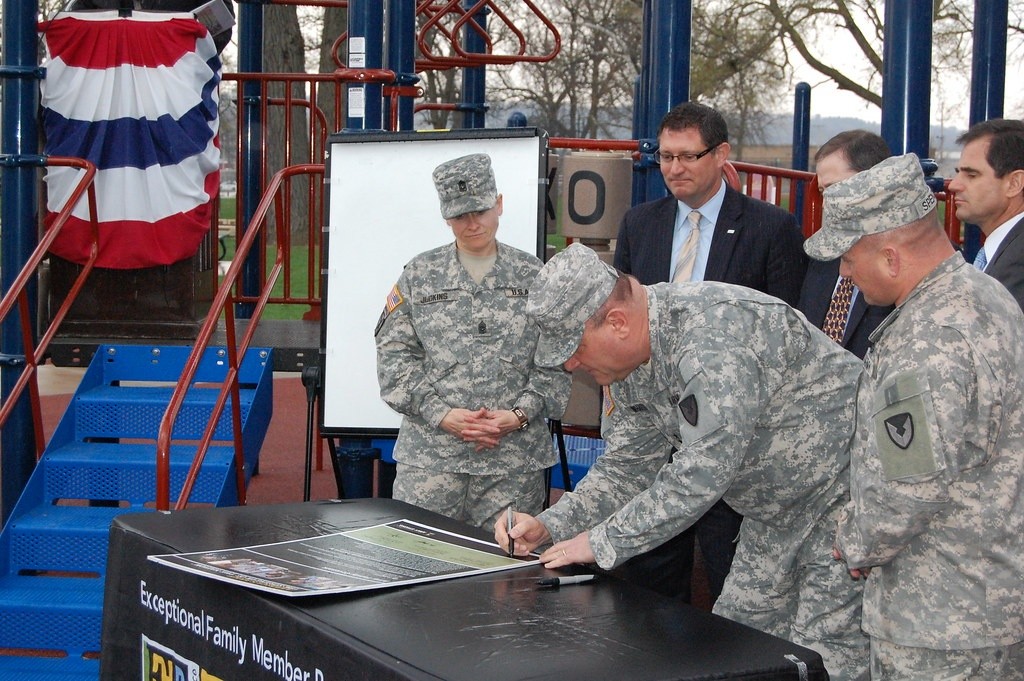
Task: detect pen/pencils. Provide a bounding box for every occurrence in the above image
[507,507,514,558]
[534,574,598,586]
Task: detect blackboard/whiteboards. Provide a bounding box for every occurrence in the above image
[321,127,549,439]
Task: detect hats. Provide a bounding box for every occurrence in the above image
[525,242,619,368]
[432,153,497,219]
[803,153,937,261]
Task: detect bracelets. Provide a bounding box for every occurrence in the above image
[509,406,530,432]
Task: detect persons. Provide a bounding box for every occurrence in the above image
[373,151,571,549]
[494,100,1024,681]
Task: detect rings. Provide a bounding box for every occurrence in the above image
[563,549,567,556]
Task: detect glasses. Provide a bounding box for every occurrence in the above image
[653,143,721,163]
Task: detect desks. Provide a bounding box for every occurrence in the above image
[96,497,829,681]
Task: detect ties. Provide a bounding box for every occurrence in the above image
[671,210,702,282]
[822,277,854,346]
[973,246,988,271]
